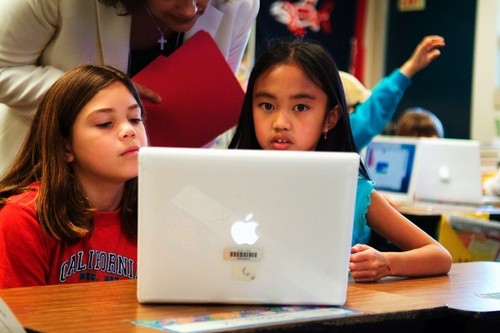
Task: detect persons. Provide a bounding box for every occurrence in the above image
[227,41,452,285]
[337,36,446,154]
[396,109,445,140]
[1,1,261,181]
[0,64,149,290]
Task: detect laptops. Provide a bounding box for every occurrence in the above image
[137,147,357,307]
[412,137,500,207]
[357,134,418,202]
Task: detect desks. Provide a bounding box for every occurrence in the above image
[0,261,500,333]
[374,188,500,262]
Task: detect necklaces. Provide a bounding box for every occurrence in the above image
[143,3,171,51]
